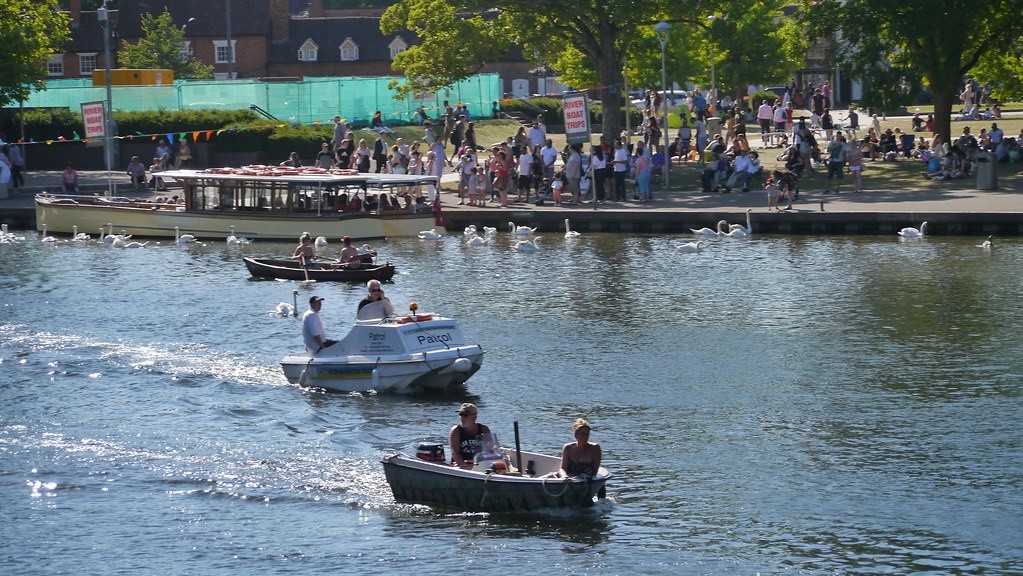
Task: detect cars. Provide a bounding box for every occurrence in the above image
[764,86,790,98]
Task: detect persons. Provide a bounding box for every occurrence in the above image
[537,417,601,480]
[356,280,394,320]
[302,296,340,354]
[0,77,1023,212]
[448,402,502,470]
[334,235,363,270]
[291,231,321,270]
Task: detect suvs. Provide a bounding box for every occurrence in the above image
[630,90,688,107]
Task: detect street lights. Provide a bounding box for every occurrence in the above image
[653,21,673,191]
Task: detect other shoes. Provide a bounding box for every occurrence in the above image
[467,202,486,208]
[784,206,792,210]
[458,202,464,205]
[922,173,930,179]
[854,189,861,193]
[823,190,829,194]
[702,186,748,192]
[489,200,493,203]
[498,204,507,207]
[524,199,529,203]
[553,193,654,206]
[514,199,520,202]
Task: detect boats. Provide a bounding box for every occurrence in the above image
[279,299,484,394]
[242,254,396,284]
[34,166,447,242]
[379,420,612,515]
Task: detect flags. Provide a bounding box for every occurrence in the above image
[433,186,445,226]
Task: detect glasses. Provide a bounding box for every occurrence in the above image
[370,289,381,292]
[459,413,475,417]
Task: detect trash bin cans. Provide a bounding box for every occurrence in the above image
[707,117,722,140]
[975,152,997,190]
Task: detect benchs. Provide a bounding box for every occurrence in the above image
[756,127,860,145]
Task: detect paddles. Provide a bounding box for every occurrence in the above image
[313,255,336,261]
[302,254,309,280]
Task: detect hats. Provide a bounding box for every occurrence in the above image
[455,403,476,414]
[963,126,970,133]
[310,296,324,303]
[766,178,773,184]
[340,236,350,241]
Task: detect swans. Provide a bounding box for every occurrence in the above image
[508,222,537,235]
[898,221,927,236]
[689,219,727,236]
[174,226,197,244]
[564,218,581,239]
[315,235,327,246]
[982,234,994,248]
[512,235,543,251]
[415,224,497,246]
[720,208,752,237]
[676,241,705,253]
[0,223,150,248]
[276,290,299,316]
[356,243,370,254]
[226,235,254,245]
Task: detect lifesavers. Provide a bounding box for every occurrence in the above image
[204,167,232,174]
[329,168,362,177]
[234,164,303,177]
[395,314,432,324]
[303,167,327,175]
